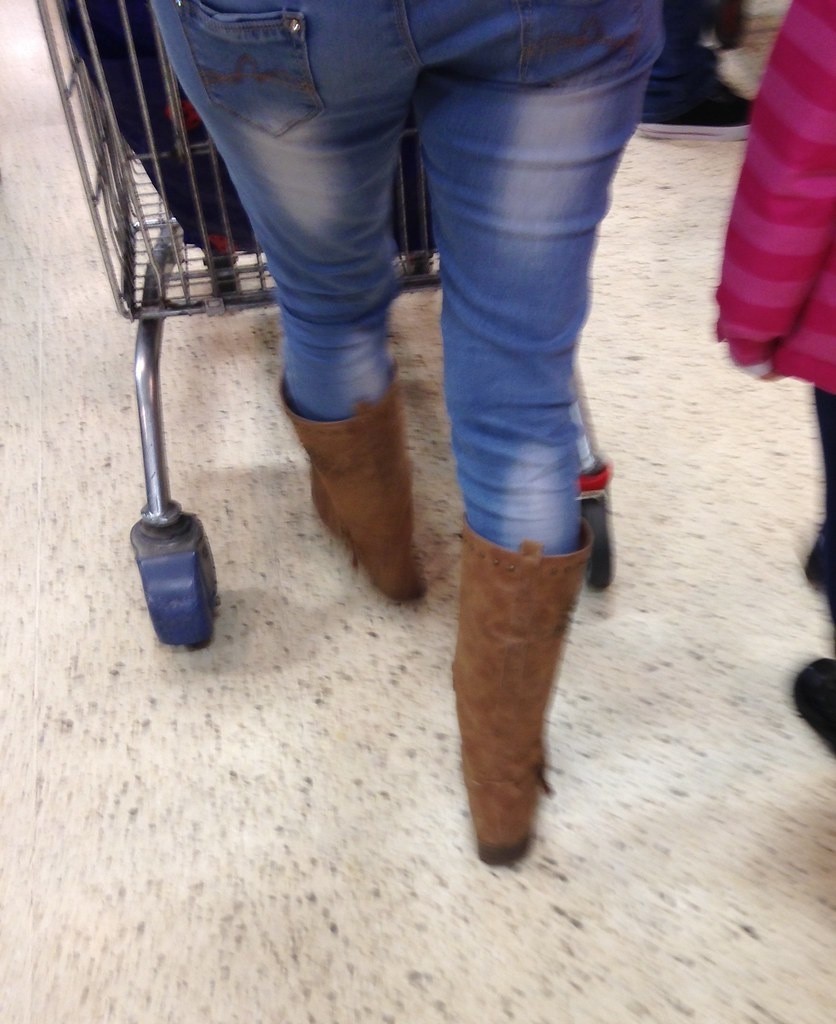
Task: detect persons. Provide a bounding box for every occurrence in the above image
[147,0,667,866]
[715,1,834,757]
[632,0,761,140]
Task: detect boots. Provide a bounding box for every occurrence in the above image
[278,361,427,602]
[452,511,595,866]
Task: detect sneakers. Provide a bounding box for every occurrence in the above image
[634,82,752,141]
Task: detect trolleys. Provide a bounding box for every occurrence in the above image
[34,1,616,639]
